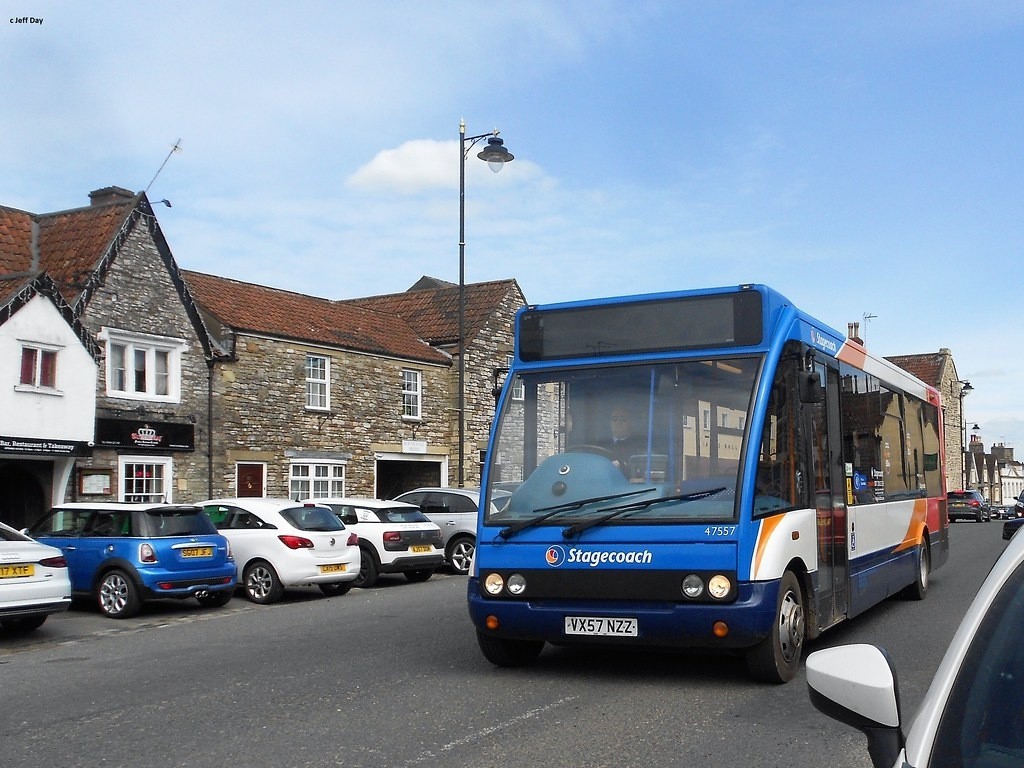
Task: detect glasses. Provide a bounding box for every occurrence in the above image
[610,416,631,422]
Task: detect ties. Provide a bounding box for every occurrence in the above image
[616,439,620,445]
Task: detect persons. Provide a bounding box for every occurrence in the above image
[590,405,656,478]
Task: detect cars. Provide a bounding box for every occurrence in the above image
[191,481,526,605]
[0,522,72,631]
[805,523,1023,768]
[18,500,239,618]
[945,489,1024,540]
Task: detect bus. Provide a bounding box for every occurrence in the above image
[466,283,950,683]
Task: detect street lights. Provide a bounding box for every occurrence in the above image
[457,118,515,490]
[959,380,976,490]
[964,418,981,450]
